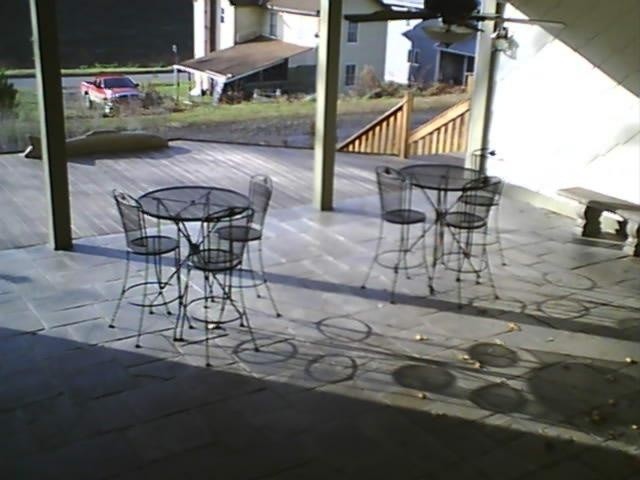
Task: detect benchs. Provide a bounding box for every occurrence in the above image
[559,187,640,255]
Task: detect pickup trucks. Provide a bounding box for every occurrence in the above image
[80,75,146,117]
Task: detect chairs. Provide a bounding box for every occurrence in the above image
[460,145,505,264]
[427,176,500,297]
[361,166,433,303]
[203,171,283,318]
[174,208,256,367]
[111,188,191,330]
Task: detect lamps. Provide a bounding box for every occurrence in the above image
[421,24,476,48]
[491,30,511,50]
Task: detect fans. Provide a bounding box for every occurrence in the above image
[345,0,566,29]
